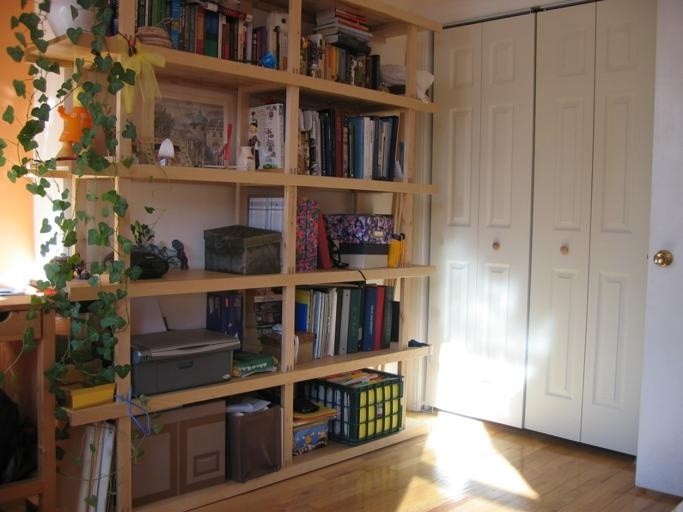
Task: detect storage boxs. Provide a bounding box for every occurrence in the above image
[327,212,397,269]
[199,220,281,276]
[115,326,283,506]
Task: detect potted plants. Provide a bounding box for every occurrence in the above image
[0,0,163,508]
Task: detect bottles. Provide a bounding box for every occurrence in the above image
[387,233,407,268]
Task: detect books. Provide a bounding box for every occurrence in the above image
[110,0,289,72]
[58,422,116,512]
[295,281,395,364]
[300,8,381,92]
[249,103,399,181]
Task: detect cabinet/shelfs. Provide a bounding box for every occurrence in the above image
[27,0,439,511]
[0,291,59,511]
